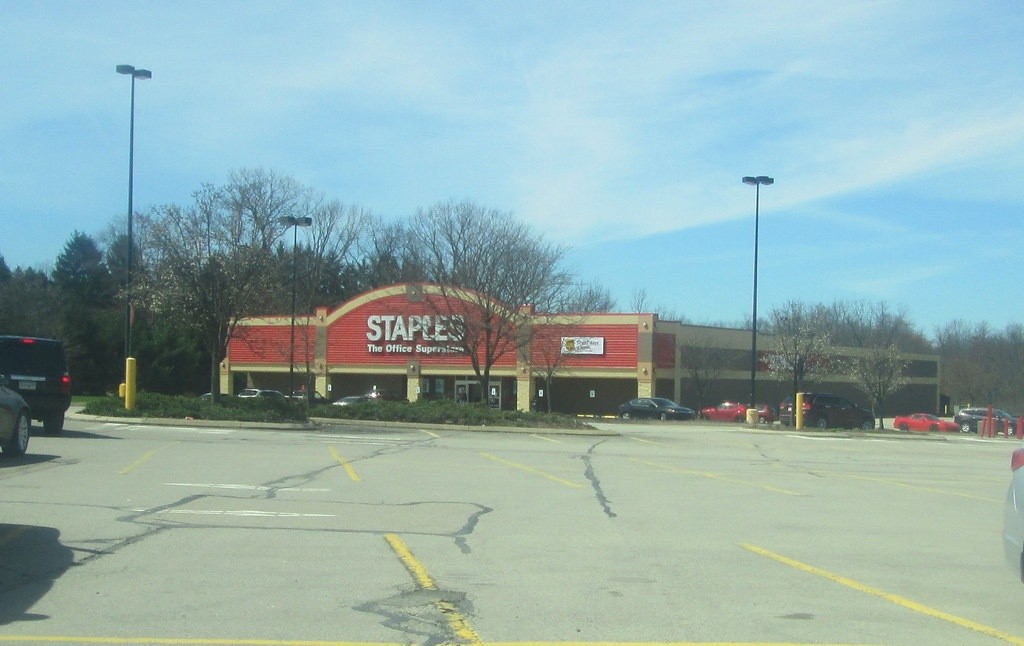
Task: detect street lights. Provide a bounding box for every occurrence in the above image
[742,176,775,408]
[116,64,152,354]
[279,216,313,400]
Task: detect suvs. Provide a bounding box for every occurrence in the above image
[779,393,876,430]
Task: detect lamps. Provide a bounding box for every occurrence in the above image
[221,362,225,367]
[643,322,646,326]
[410,364,414,370]
[655,322,659,328]
[319,315,322,320]
[641,368,647,373]
[521,367,525,372]
[319,363,323,368]
[412,291,415,295]
[654,367,657,372]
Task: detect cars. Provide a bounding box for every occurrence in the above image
[953,408,1019,435]
[0,335,72,433]
[697,401,749,423]
[332,397,372,406]
[618,397,696,421]
[238,389,285,397]
[366,390,392,400]
[893,414,960,433]
[285,390,326,399]
[0,374,32,456]
[748,403,779,424]
